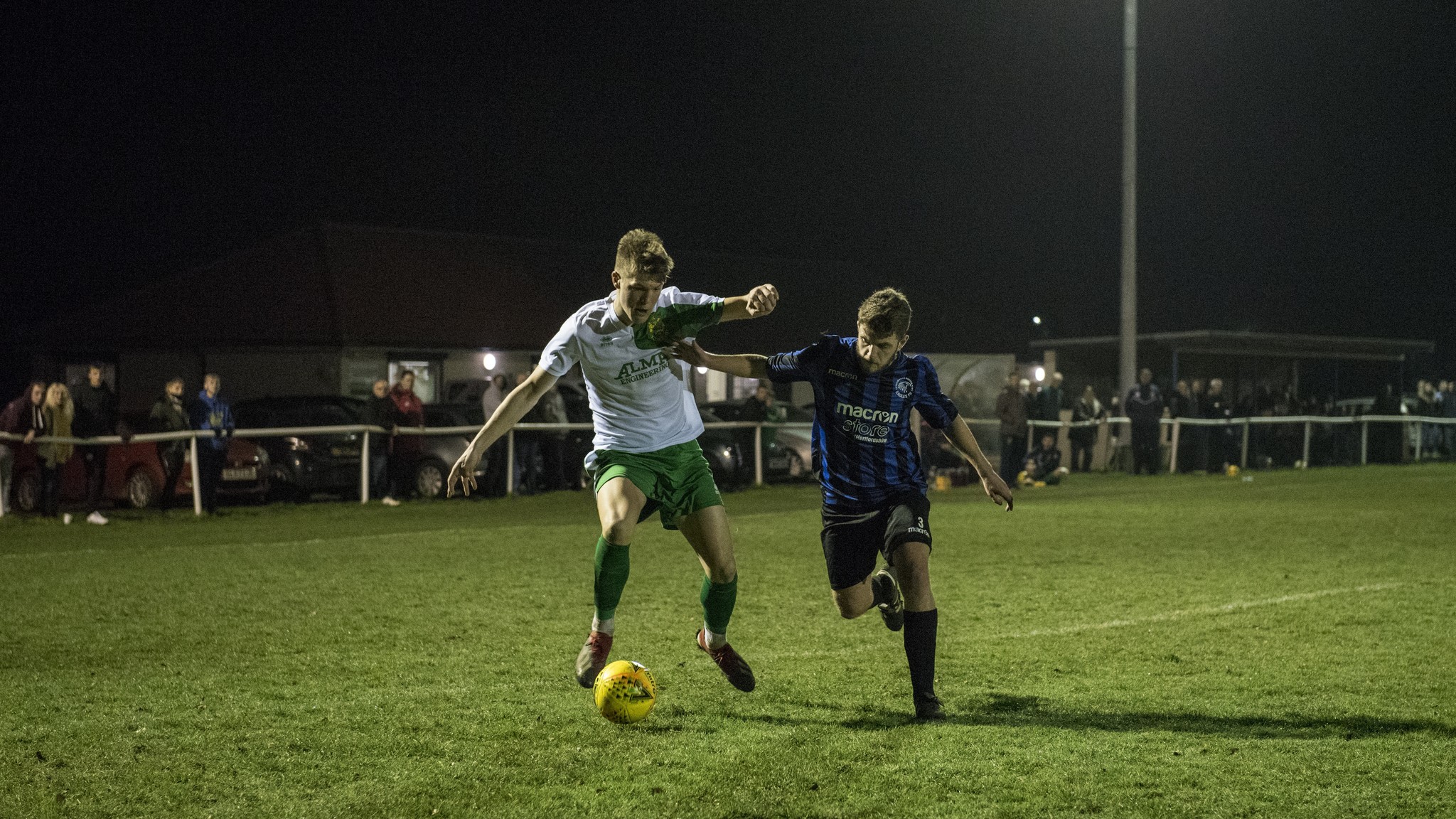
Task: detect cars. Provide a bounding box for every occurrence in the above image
[451,377,817,491]
[3,395,490,502]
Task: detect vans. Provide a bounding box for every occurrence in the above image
[1325,399,1423,460]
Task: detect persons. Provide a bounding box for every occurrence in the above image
[1124,368,1164,475]
[673,288,1016,727]
[541,385,572,491]
[997,371,1069,486]
[1235,385,1403,466]
[364,380,402,506]
[482,371,512,495]
[1067,385,1106,473]
[0,380,48,512]
[389,371,425,500]
[32,382,76,517]
[1415,380,1456,458]
[1167,379,1231,474]
[70,362,131,526]
[447,228,780,692]
[193,373,236,509]
[734,387,775,487]
[510,372,546,493]
[149,379,195,510]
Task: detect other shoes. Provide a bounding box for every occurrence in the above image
[382,496,400,506]
[63,513,73,525]
[86,511,109,525]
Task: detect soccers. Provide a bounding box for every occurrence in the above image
[592,660,657,724]
[1225,465,1240,478]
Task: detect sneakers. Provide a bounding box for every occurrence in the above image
[877,565,903,632]
[698,629,755,692]
[576,631,613,689]
[914,695,946,721]
